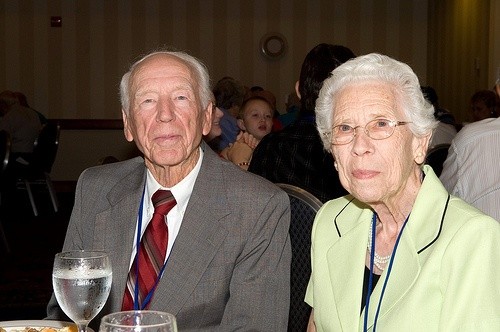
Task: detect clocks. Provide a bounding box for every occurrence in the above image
[259,31,290,61]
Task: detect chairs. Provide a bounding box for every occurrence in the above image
[10,119,63,217]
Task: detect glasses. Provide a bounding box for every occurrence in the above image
[324,119,412,146]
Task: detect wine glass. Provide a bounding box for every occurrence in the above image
[51,250,113,332]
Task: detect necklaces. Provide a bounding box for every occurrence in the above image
[367,219,391,272]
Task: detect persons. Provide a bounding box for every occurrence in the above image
[203,91,260,172]
[439,79,500,223]
[237,97,274,141]
[246,43,357,205]
[304,53,499,332]
[469,90,499,122]
[422,86,457,150]
[208,80,243,156]
[45,53,291,331]
[217,75,282,130]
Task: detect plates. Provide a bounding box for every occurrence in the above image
[0,320,95,332]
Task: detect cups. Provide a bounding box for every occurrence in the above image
[99,310,178,332]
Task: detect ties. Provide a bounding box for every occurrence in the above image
[120,191,177,331]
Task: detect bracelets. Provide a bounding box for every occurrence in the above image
[236,162,250,167]
